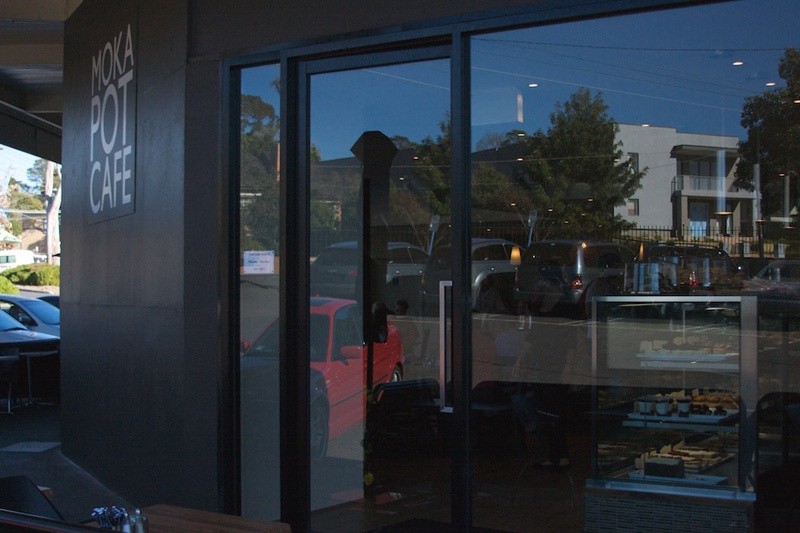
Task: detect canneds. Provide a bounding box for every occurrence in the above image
[637,396,690,418]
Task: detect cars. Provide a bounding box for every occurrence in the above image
[0,294,61,337]
[1,309,62,395]
[737,260,800,317]
[241,297,406,467]
[37,295,61,311]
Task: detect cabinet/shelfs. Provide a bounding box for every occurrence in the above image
[580,296,758,508]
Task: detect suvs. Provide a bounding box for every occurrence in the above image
[469,236,527,315]
[633,241,737,294]
[514,238,638,321]
[308,241,435,312]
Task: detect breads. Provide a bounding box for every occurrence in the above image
[636,432,738,480]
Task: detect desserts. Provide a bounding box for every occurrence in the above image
[668,378,780,418]
[636,332,794,354]
